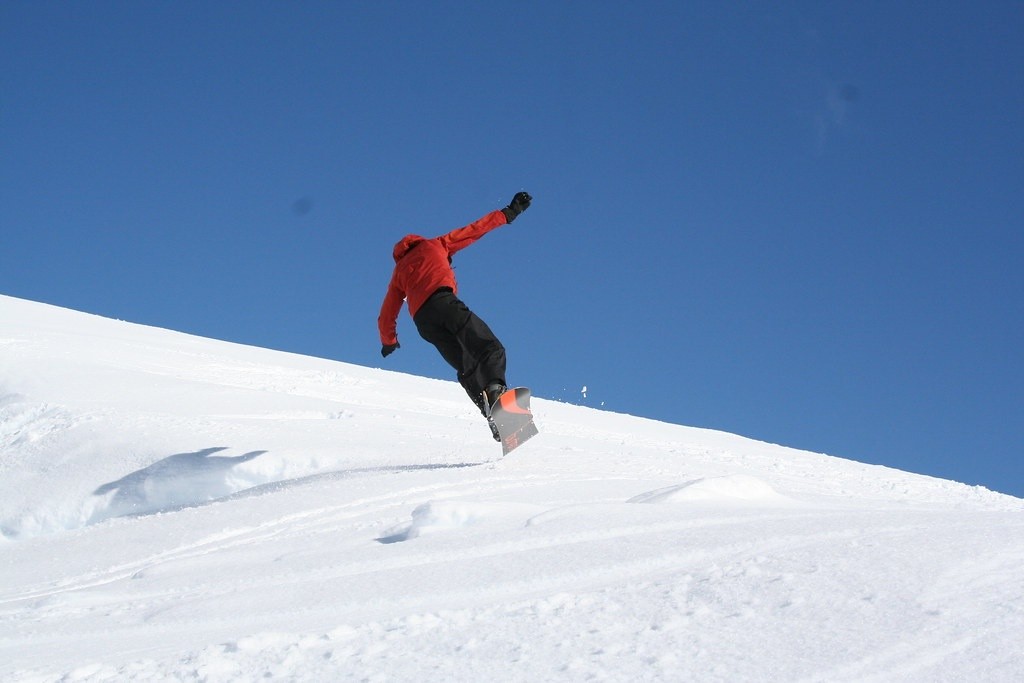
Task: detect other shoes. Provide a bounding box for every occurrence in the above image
[484,383,505,409]
[487,415,501,442]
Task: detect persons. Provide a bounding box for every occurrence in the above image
[377,192,533,441]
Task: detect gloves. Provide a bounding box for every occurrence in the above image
[501,192,533,224]
[381,341,400,358]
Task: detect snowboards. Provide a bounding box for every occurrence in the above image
[489,386,539,458]
